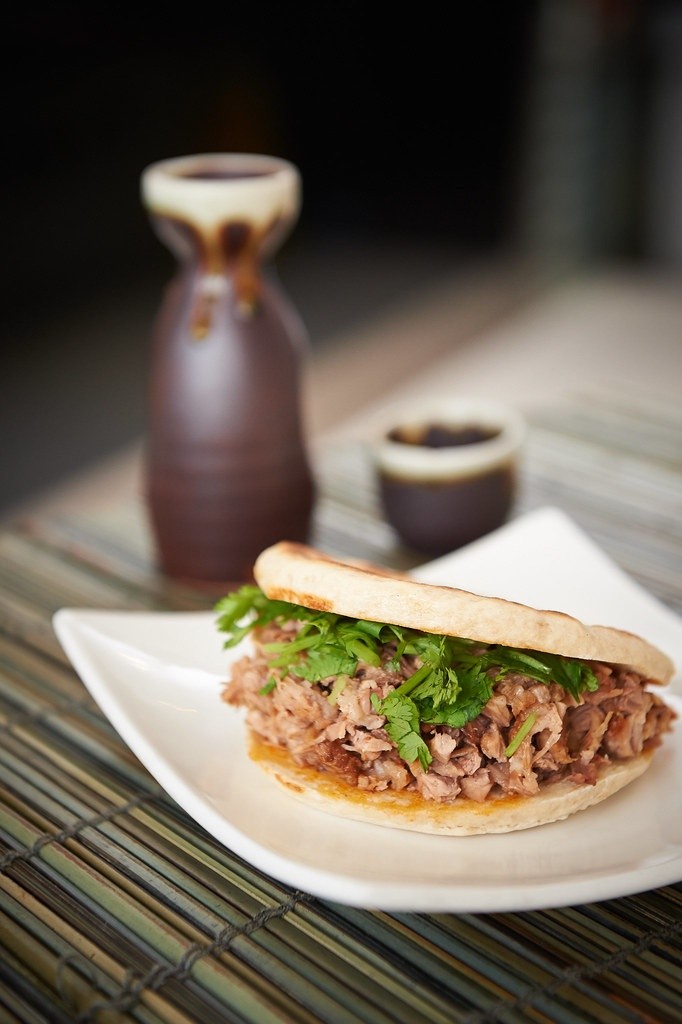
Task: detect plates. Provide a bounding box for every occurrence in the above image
[51,505,682,914]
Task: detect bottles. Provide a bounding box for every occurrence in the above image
[138,150,322,584]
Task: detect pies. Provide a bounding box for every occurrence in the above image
[216,540,678,836]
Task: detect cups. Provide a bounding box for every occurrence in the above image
[367,403,532,556]
